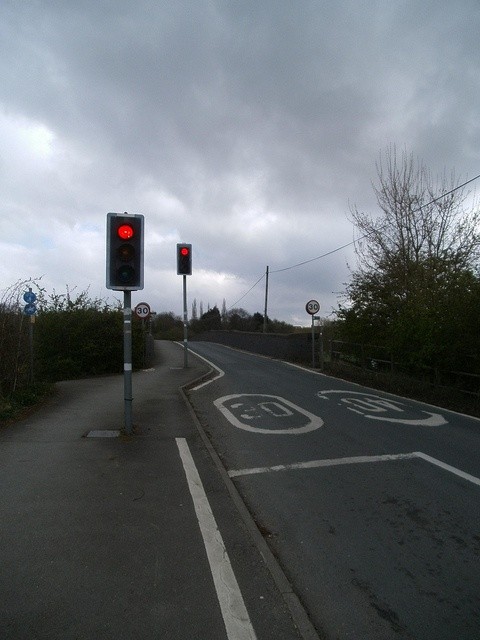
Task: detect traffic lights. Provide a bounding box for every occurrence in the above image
[177,243,192,274]
[106,213,143,290]
